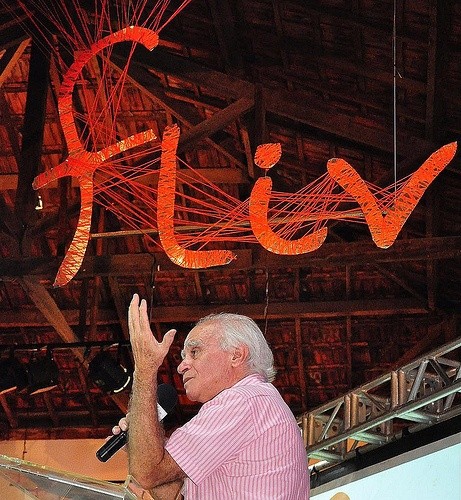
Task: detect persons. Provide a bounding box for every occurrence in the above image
[106,292,310,499]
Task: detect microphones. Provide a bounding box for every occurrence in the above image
[97,383,179,463]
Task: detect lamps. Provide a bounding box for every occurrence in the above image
[88,348,132,394]
[0,356,24,394]
[27,356,59,395]
